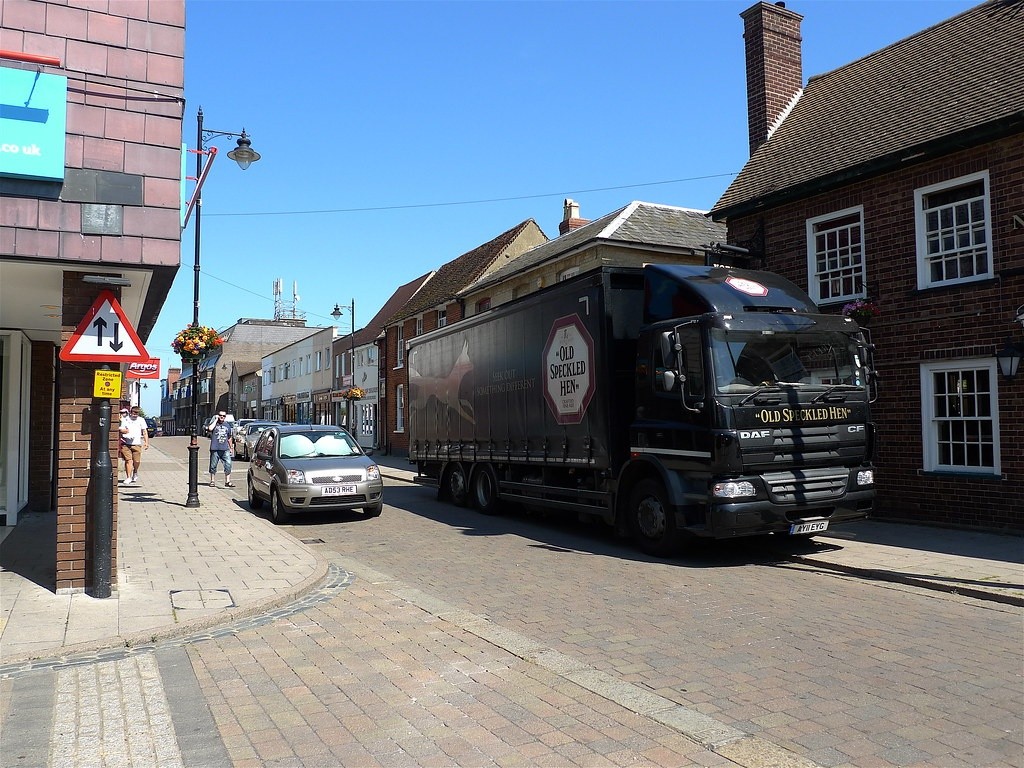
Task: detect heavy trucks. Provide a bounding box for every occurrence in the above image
[405,242,883,561]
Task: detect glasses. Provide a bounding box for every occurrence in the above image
[219,414,226,417]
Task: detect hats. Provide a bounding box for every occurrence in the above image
[120,408,128,413]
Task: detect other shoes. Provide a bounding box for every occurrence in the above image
[123,478,131,484]
[131,476,138,481]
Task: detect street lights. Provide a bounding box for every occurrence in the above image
[185,106,262,509]
[136,378,148,407]
[330,297,355,440]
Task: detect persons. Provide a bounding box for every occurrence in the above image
[118,406,150,485]
[208,412,238,489]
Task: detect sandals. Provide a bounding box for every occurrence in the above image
[210,482,215,486]
[225,482,235,487]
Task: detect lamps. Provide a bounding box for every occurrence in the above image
[996,338,1023,381]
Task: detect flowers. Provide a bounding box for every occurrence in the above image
[170,325,223,354]
[341,387,366,400]
[840,301,883,319]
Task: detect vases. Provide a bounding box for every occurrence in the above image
[350,397,360,401]
[182,353,204,359]
[857,315,872,326]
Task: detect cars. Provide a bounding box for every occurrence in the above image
[201,414,299,462]
[142,418,157,438]
[246,424,384,524]
